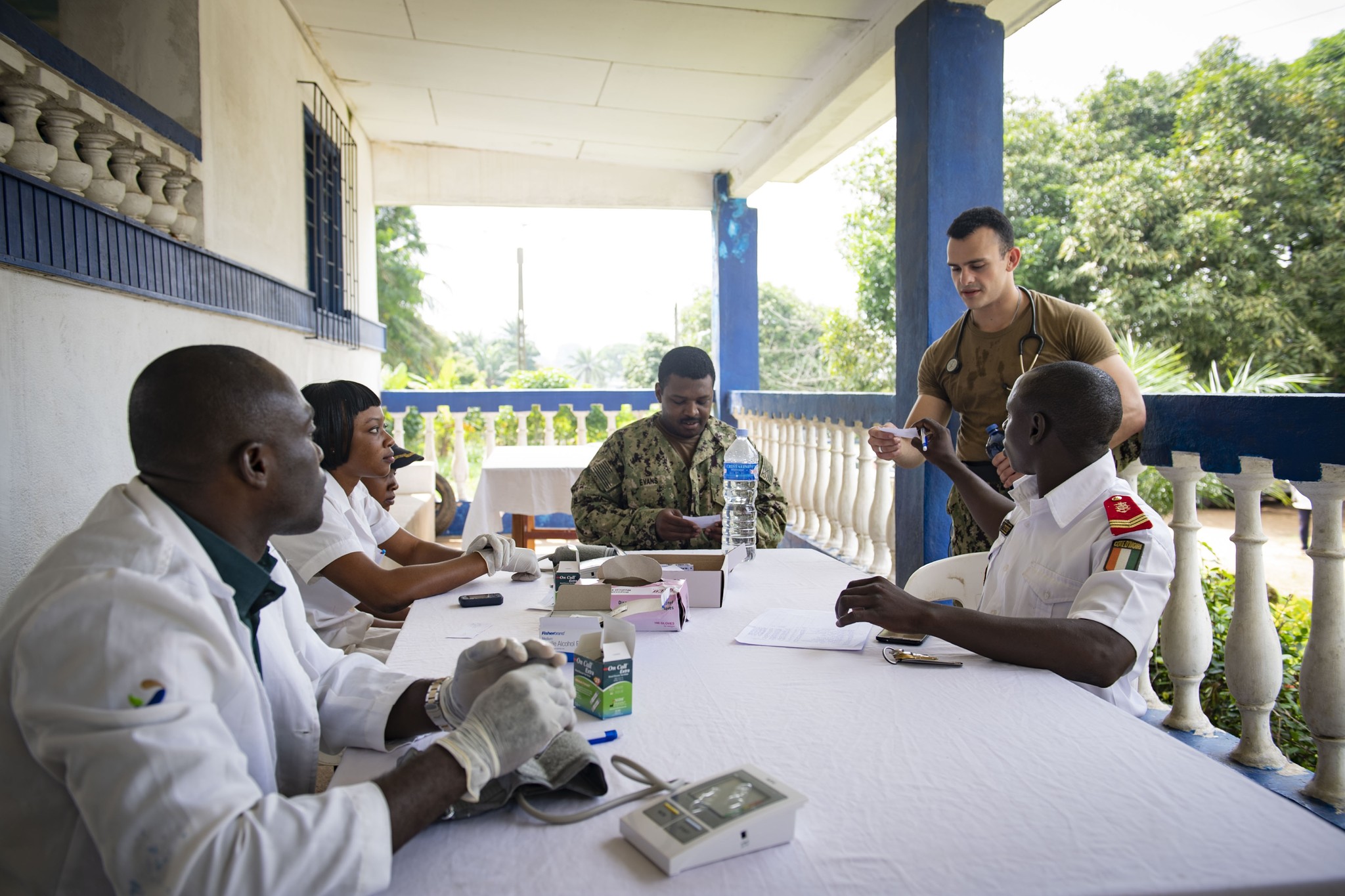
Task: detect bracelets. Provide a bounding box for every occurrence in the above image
[424,675,457,732]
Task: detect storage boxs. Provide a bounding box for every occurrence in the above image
[623,541,748,610]
[535,579,622,664]
[568,612,644,720]
[551,543,582,602]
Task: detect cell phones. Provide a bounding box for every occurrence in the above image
[875,628,928,646]
[458,592,503,607]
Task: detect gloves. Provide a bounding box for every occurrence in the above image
[461,532,516,569]
[441,636,567,730]
[435,665,576,802]
[473,547,542,582]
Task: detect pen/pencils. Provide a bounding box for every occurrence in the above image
[920,427,929,450]
[583,729,622,745]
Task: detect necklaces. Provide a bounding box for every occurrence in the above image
[970,286,1022,327]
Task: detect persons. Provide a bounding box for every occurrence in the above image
[868,207,1146,556]
[353,442,424,629]
[268,379,542,664]
[1290,485,1312,550]
[834,362,1176,718]
[570,346,788,551]
[0,344,578,896]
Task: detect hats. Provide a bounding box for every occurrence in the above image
[389,443,424,469]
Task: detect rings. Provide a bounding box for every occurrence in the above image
[878,446,883,453]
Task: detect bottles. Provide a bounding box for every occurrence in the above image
[985,424,1014,491]
[721,429,759,563]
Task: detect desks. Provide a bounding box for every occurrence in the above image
[298,545,1345,896]
[459,441,605,550]
